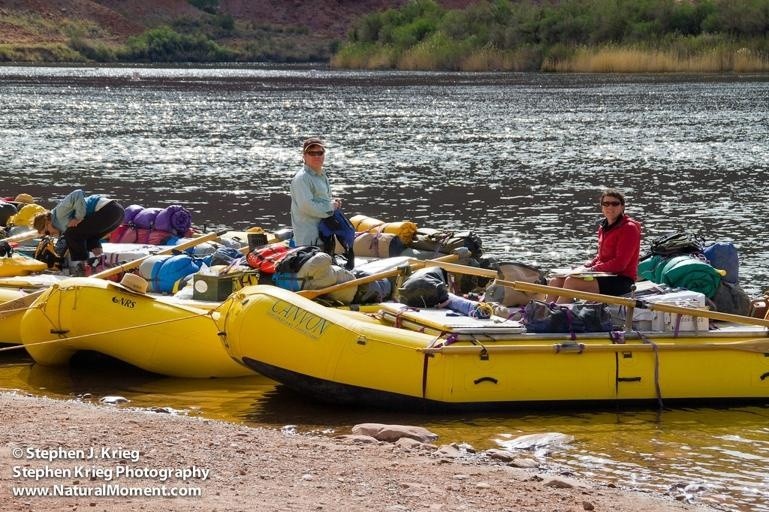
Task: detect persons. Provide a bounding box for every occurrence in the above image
[33,188,125,277]
[544,189,642,305]
[290,138,343,252]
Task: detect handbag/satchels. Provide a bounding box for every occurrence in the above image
[483,262,547,307]
[397,267,449,308]
[525,299,613,333]
[651,232,701,255]
[318,208,355,249]
[706,242,738,282]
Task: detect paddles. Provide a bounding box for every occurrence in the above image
[1,230,227,318]
[296,250,471,300]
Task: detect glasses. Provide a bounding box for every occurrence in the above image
[44,224,51,235]
[601,200,621,207]
[306,149,325,156]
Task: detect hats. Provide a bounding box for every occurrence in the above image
[303,138,324,151]
[6,193,35,205]
[33,214,46,234]
[106,272,157,299]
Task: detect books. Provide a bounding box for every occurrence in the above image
[550,266,618,279]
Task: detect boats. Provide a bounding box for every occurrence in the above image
[216,282,766,412]
[0,181,208,353]
[20,216,446,376]
